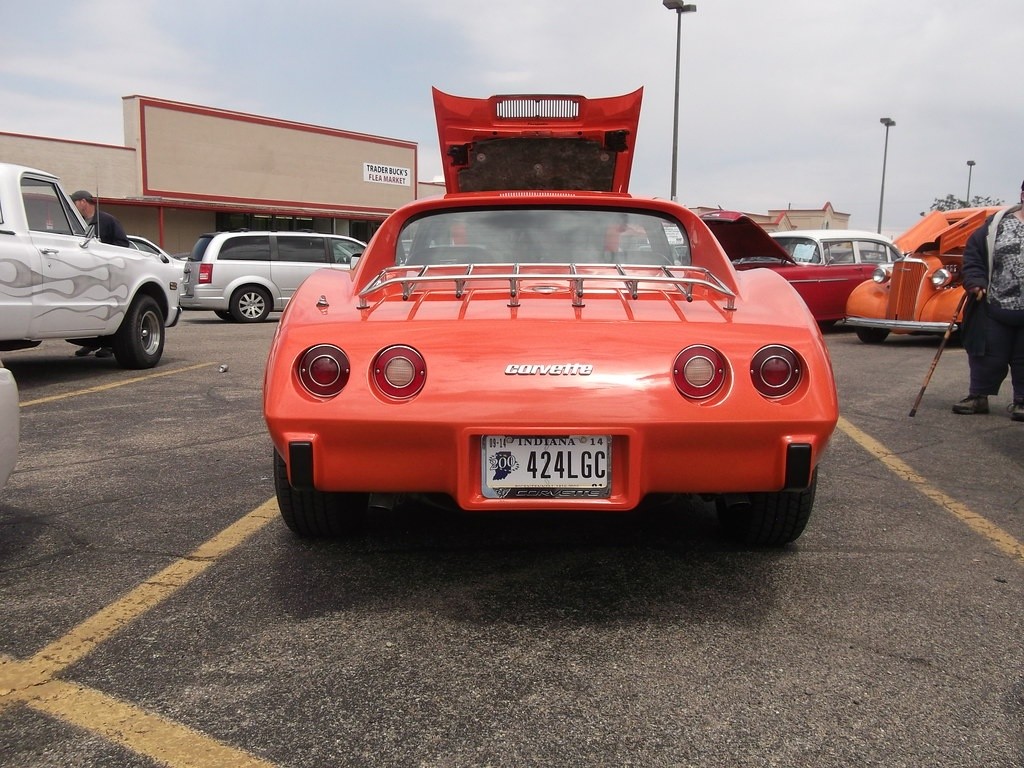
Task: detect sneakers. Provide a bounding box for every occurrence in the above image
[1006,397,1024,421]
[952,394,989,415]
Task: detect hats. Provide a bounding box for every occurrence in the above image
[69,190,93,202]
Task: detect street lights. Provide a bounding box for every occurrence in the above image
[661,0,698,201]
[964,160,976,207]
[876,117,896,232]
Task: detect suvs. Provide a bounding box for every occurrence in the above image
[1,161,184,371]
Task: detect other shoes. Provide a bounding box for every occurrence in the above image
[75,346,100,356]
[95,347,114,358]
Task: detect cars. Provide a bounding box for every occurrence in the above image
[126,233,188,299]
[400,239,497,264]
[698,205,1013,344]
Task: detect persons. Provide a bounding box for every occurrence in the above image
[71,190,130,357]
[953,179,1024,421]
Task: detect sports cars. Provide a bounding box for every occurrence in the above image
[264,85,840,549]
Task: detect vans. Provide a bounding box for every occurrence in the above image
[178,227,368,324]
